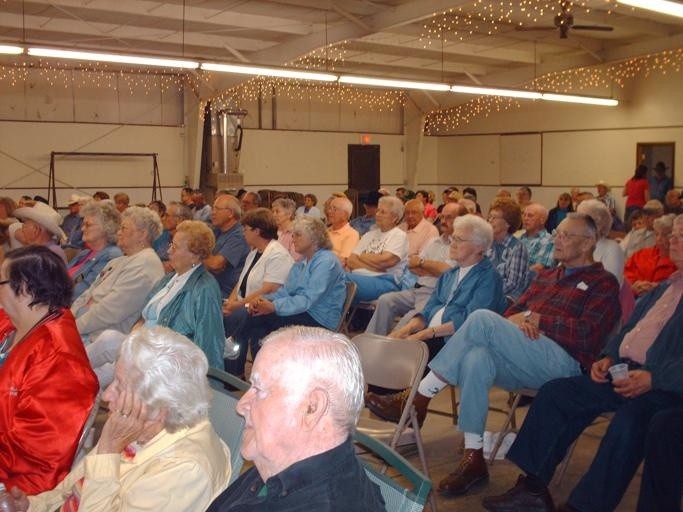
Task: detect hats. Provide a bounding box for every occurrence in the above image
[68,195,80,205]
[14,202,68,241]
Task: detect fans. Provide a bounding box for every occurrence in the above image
[515,0,613,39]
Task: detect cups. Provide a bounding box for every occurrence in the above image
[607,363,628,381]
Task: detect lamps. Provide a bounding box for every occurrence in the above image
[0,1,618,106]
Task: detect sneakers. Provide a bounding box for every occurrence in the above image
[222,337,241,360]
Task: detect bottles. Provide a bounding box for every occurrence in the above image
[0,483,17,512]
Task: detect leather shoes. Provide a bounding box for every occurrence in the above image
[364,386,433,431]
[483,474,553,511]
[438,448,489,497]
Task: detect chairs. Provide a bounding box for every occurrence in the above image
[60,243,579,512]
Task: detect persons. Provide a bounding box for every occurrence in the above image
[1,160,683,510]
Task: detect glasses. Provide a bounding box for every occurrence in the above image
[552,229,590,241]
[448,235,474,243]
